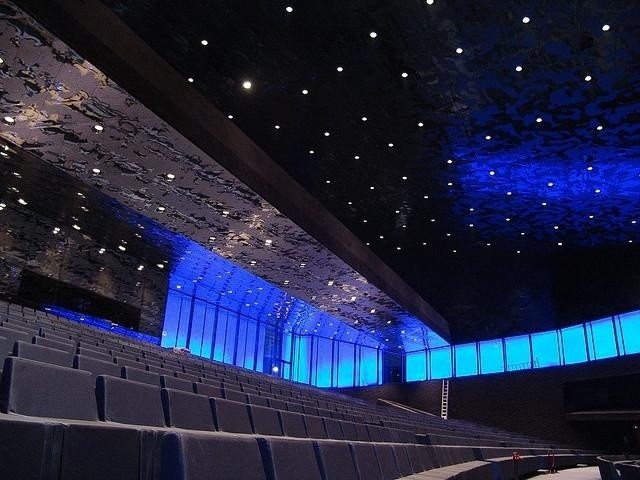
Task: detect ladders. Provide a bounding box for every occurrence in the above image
[440,379,449,420]
